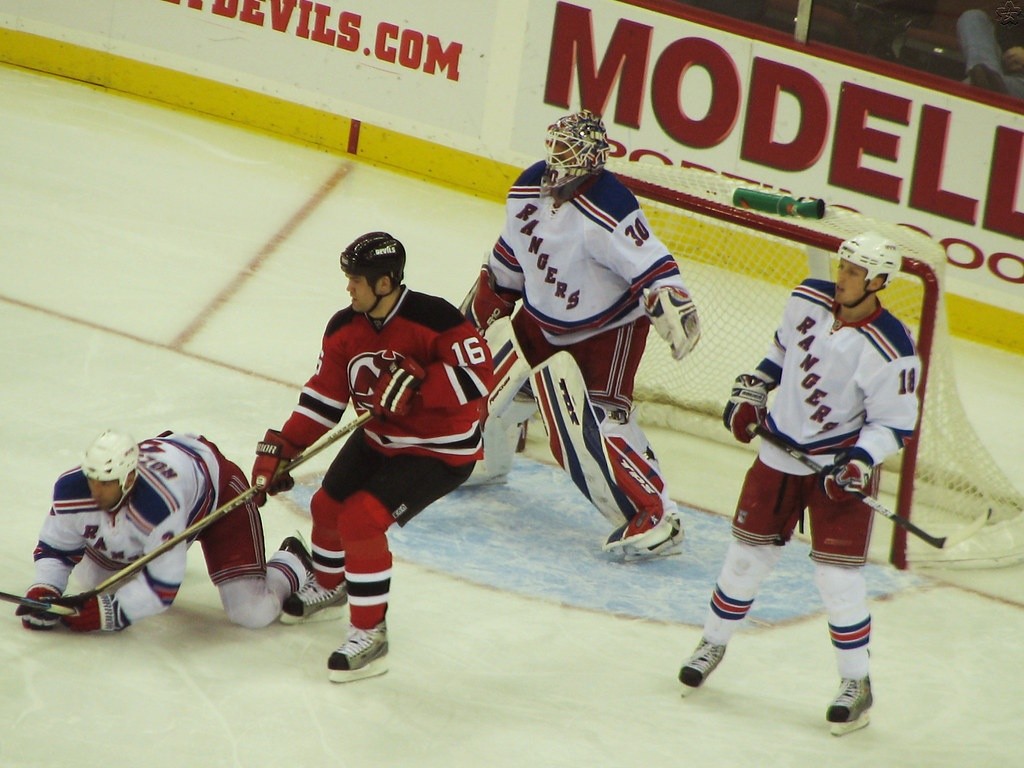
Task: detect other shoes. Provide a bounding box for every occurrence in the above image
[967,63,1007,96]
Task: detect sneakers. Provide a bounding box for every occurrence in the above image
[680,636,726,697]
[825,675,873,736]
[628,518,683,561]
[280,569,348,626]
[327,620,389,682]
[281,532,313,576]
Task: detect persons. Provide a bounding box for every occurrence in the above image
[747,0,1024,95]
[16,429,312,633]
[678,231,923,724]
[458,112,698,553]
[253,231,493,669]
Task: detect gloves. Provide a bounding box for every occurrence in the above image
[14,581,65,629]
[644,287,700,359]
[818,447,873,502]
[248,429,301,507]
[58,592,129,632]
[372,362,425,417]
[722,370,768,444]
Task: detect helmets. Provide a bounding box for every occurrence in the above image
[541,110,611,190]
[837,232,902,287]
[81,430,140,482]
[340,232,406,286]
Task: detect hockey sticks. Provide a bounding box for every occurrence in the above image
[1,591,83,618]
[36,412,373,607]
[747,424,994,553]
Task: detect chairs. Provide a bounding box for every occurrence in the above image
[760,0,992,78]
